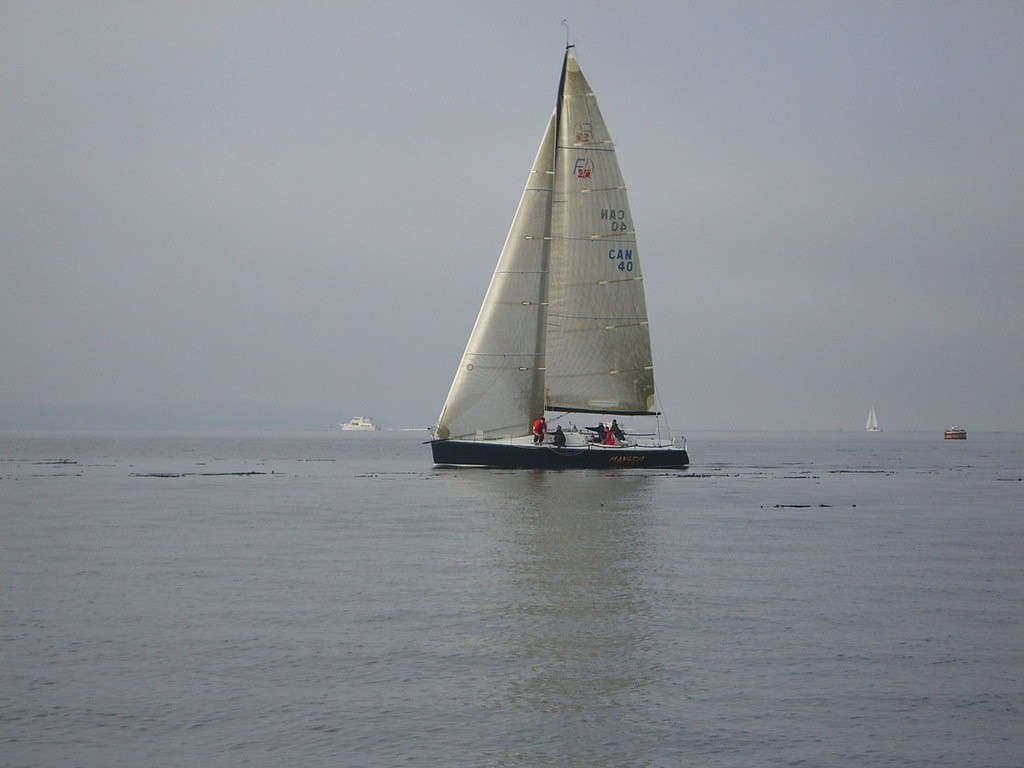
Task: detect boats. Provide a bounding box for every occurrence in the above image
[341,416,383,432]
[943,425,969,440]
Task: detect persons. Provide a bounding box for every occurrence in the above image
[531,417,548,446]
[600,426,615,446]
[546,424,566,448]
[611,419,627,442]
[585,422,607,443]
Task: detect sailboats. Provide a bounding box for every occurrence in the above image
[424,36,691,472]
[864,407,879,432]
